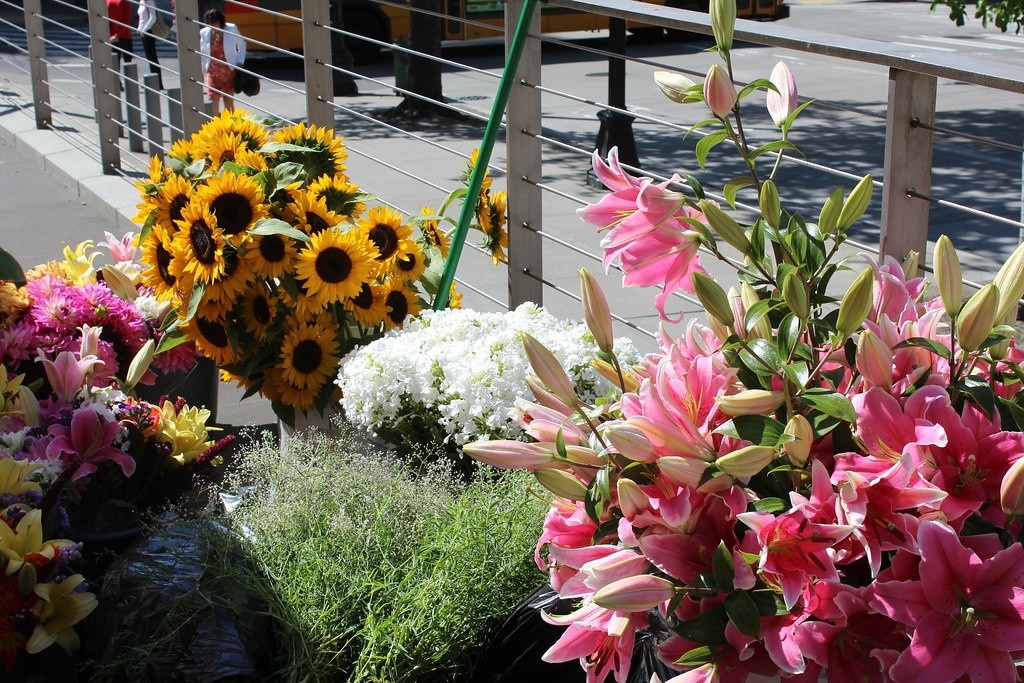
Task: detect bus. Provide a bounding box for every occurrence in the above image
[172,0,791,65]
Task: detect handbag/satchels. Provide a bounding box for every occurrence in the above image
[146,0,169,42]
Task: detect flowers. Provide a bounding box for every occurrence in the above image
[0,0,1024,683]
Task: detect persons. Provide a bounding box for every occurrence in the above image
[137,0,164,90]
[199,9,246,117]
[107,0,133,91]
